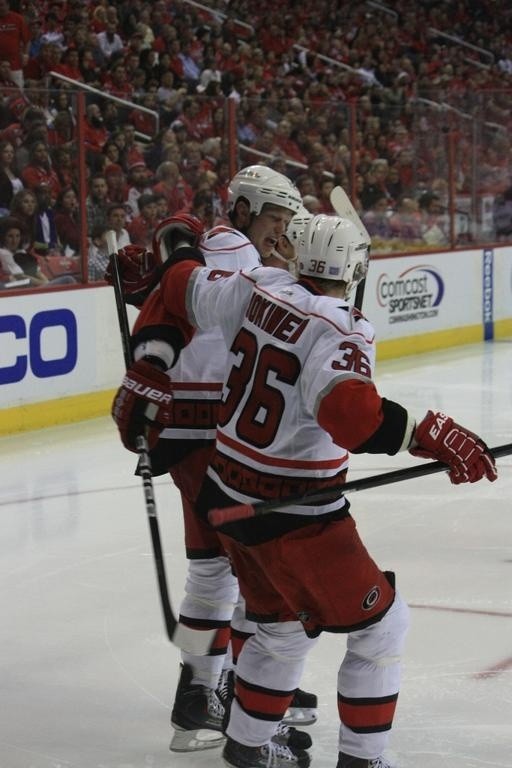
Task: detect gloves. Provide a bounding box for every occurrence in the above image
[409,410,497,487]
[110,356,173,454]
[105,244,157,295]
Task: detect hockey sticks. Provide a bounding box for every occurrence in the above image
[104,230,218,656]
[330,185,371,320]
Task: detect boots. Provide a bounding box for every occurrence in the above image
[223,741,310,768]
[334,752,394,768]
[170,663,231,734]
[288,688,318,708]
[271,724,313,750]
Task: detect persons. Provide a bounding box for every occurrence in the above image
[152,208,499,766]
[103,204,321,714]
[1,1,512,291]
[111,162,320,754]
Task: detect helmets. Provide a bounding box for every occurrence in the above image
[296,214,368,294]
[224,164,304,214]
[272,205,314,264]
